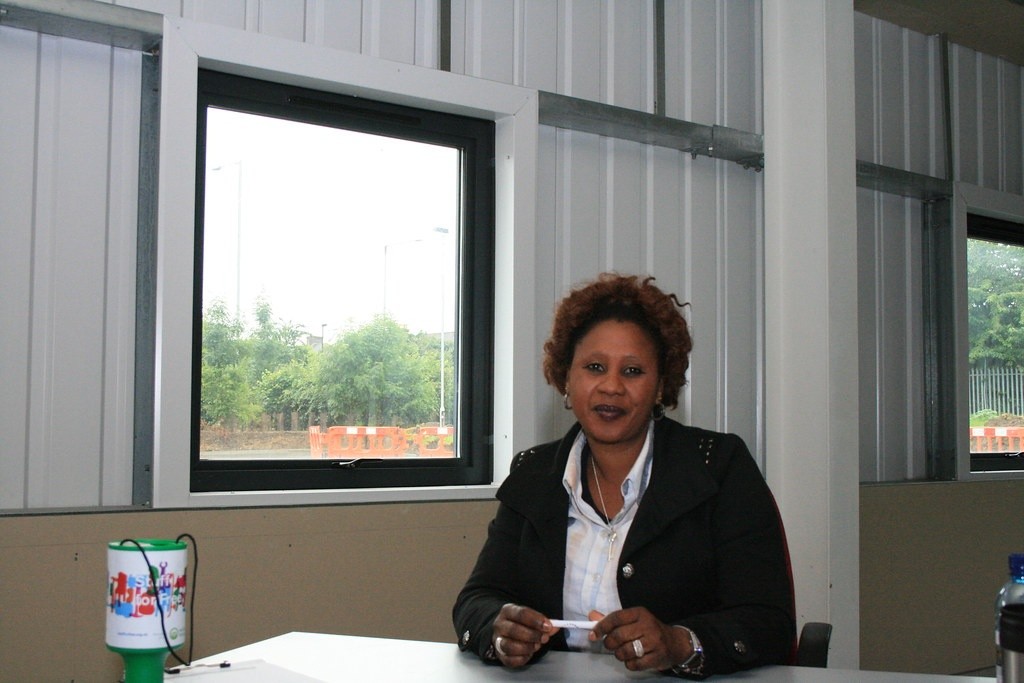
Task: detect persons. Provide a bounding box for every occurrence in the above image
[453,275,799,680]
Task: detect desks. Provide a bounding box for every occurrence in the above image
[164,631,996,683]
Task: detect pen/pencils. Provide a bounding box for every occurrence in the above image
[548,618,598,630]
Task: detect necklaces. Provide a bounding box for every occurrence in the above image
[589,452,645,561]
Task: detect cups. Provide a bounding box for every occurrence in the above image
[998,603,1024,683]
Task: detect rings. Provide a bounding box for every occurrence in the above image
[633,638,644,657]
[494,637,505,656]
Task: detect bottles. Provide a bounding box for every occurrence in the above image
[995,553,1024,683]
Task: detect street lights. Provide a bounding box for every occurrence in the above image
[322,325,326,352]
[434,227,448,432]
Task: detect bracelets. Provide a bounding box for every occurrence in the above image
[671,628,706,678]
